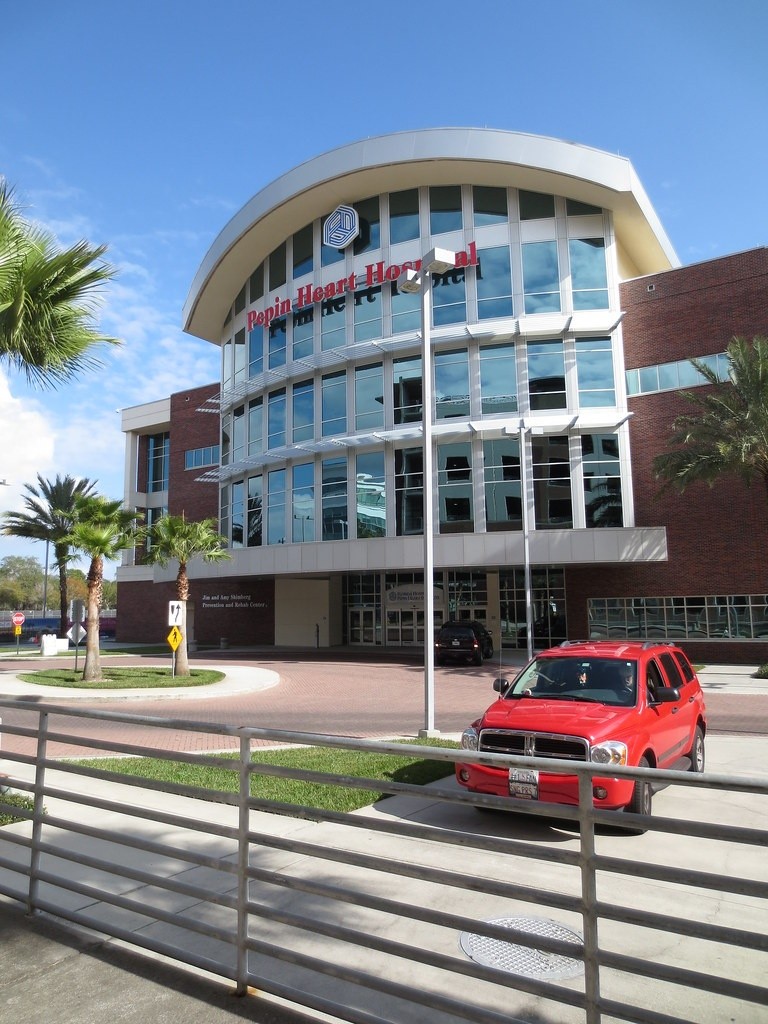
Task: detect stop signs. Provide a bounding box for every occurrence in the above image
[13,614,25,626]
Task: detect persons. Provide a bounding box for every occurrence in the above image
[575,664,655,704]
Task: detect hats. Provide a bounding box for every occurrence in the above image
[618,663,633,677]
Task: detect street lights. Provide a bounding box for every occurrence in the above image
[501,426,543,662]
[396,246,455,740]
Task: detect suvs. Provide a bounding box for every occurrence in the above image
[456,639,704,834]
[436,620,494,666]
